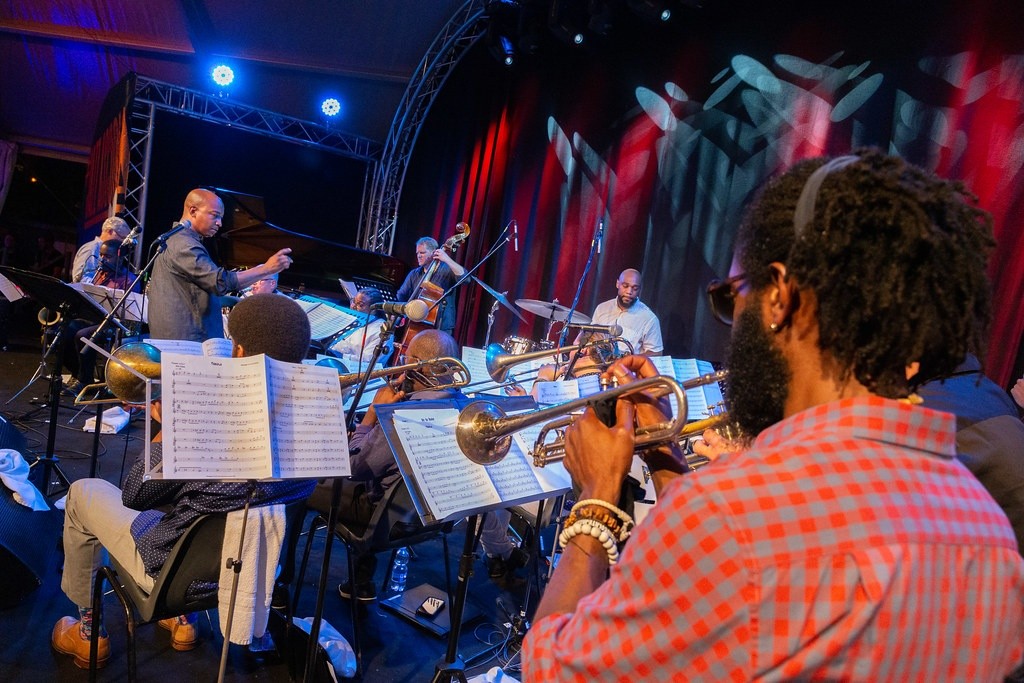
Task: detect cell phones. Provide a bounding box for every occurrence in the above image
[416,597,445,618]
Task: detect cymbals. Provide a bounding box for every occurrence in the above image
[470,275,530,326]
[514,297,593,326]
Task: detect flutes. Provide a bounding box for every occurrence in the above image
[678,411,735,441]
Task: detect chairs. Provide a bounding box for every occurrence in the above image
[89,500,305,683]
[292,476,454,680]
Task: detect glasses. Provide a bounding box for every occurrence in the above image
[111,229,126,241]
[705,264,777,327]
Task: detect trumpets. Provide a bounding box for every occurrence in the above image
[455,368,731,470]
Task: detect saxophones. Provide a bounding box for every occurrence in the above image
[37,266,105,354]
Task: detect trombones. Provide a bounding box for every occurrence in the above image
[460,336,637,398]
[312,357,472,413]
[73,341,163,406]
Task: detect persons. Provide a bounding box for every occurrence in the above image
[51,144,1024,683]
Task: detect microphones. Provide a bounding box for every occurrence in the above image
[597,218,604,253]
[370,300,428,323]
[119,226,142,248]
[153,220,192,244]
[567,322,623,336]
[514,221,519,251]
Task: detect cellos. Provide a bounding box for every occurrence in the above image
[386,221,472,385]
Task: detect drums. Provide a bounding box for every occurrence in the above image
[501,335,542,355]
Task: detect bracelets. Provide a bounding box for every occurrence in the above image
[572,519,617,544]
[558,524,619,566]
[562,509,621,536]
[570,498,636,542]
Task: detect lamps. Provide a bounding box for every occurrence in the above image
[487,0,672,66]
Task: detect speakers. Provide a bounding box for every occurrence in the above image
[0,414,29,458]
[0,475,64,606]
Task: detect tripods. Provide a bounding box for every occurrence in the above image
[0,266,127,498]
[376,393,575,683]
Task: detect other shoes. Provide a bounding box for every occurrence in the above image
[510,548,529,567]
[61,377,94,396]
[339,579,378,601]
[480,552,503,578]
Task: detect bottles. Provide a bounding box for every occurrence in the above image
[507,536,521,548]
[391,546,409,591]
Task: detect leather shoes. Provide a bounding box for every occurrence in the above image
[158,615,198,651]
[51,616,112,670]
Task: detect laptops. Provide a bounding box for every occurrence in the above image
[379,582,484,638]
